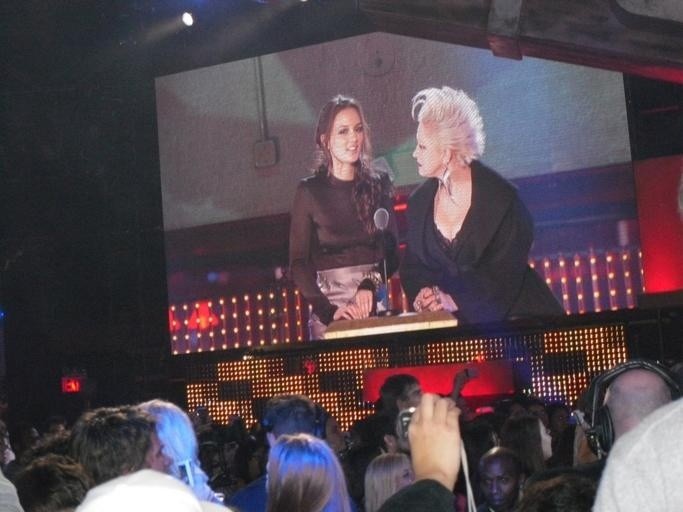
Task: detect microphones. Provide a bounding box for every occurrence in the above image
[373,207,401,316]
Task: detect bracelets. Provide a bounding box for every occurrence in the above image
[362,273,380,292]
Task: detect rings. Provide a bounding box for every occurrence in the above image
[435,293,441,304]
[433,285,439,294]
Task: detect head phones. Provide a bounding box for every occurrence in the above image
[576,355,683,460]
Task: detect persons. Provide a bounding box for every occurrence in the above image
[0,358,683,512]
[395,84,568,324]
[285,92,401,340]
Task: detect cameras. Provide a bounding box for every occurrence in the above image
[465,368,477,378]
[394,407,414,441]
